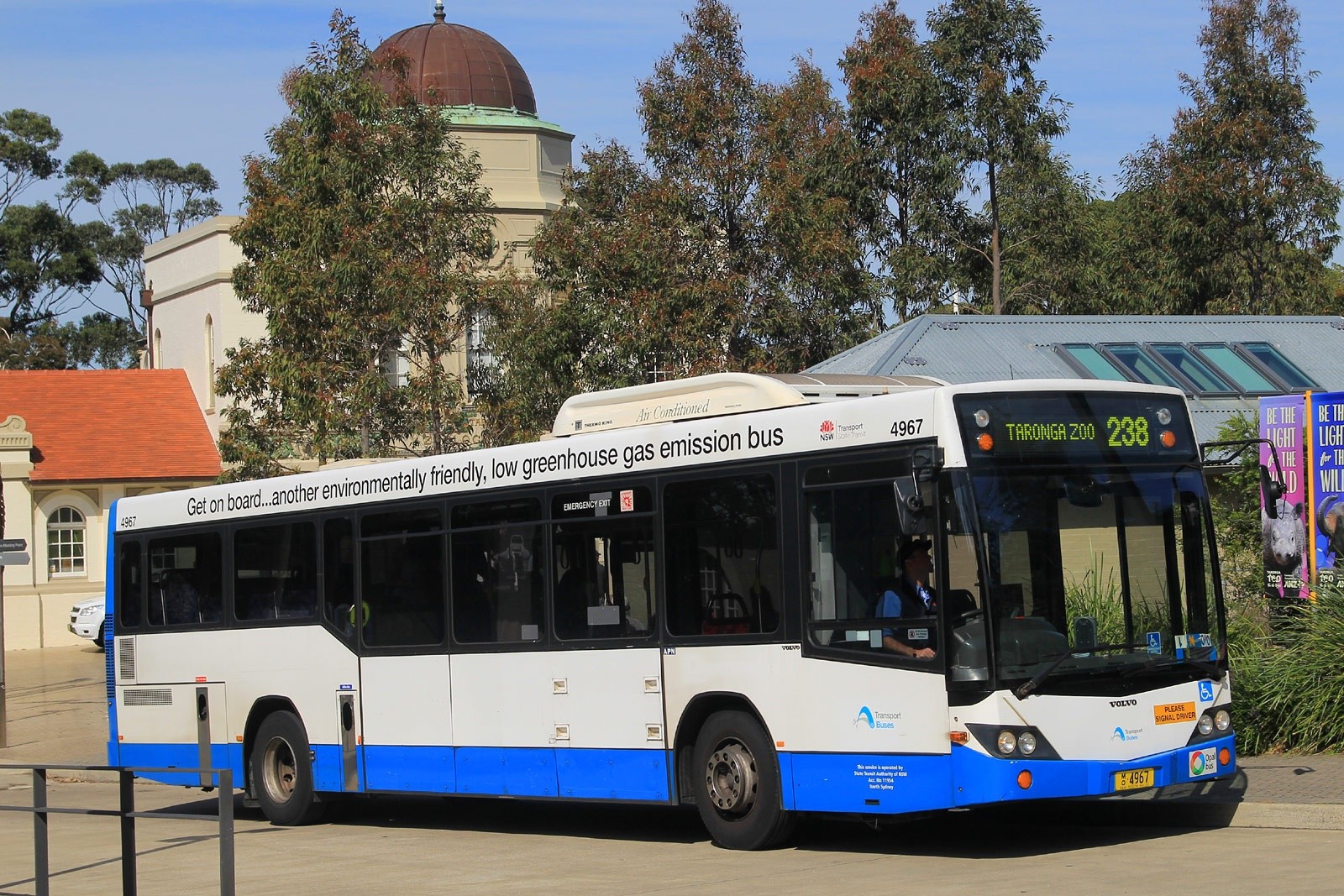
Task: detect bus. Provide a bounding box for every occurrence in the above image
[102,373,1290,852]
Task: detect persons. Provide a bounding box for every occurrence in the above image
[876,538,956,660]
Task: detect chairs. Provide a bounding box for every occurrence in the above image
[945,588,977,616]
[156,568,381,646]
[849,553,881,639]
[699,592,755,636]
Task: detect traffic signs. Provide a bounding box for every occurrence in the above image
[0,538,27,552]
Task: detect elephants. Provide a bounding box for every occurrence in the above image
[1317,491,1344,571]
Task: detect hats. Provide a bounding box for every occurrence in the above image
[896,539,932,566]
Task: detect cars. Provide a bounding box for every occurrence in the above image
[67,596,106,648]
